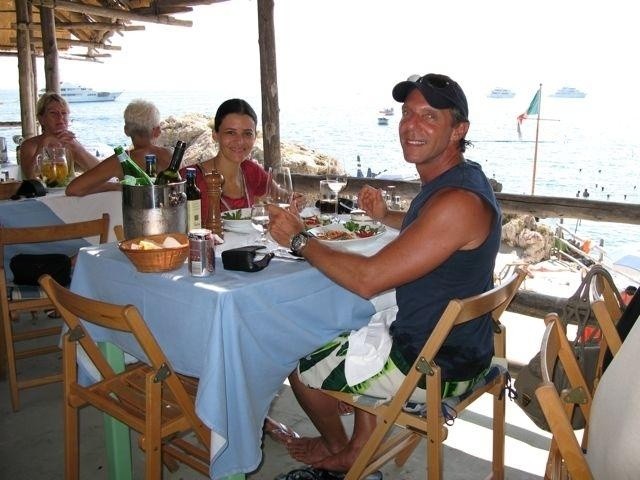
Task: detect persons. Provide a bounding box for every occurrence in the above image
[63,99,185,199]
[178,98,309,446]
[18,91,103,319]
[264,72,502,472]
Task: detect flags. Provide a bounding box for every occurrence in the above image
[513,84,541,141]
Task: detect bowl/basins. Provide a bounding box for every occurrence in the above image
[350,211,380,228]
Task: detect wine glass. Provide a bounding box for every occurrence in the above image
[326,163,348,213]
[249,206,275,247]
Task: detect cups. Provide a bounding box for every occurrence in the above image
[266,166,294,209]
[320,178,338,215]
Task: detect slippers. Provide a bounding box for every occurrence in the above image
[277,465,383,480]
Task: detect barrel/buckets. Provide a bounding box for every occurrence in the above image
[119,177,190,244]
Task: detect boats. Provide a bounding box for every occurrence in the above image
[378,118,387,126]
[384,108,393,117]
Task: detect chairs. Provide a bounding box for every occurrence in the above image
[586,265,631,399]
[1,214,110,413]
[533,313,598,479]
[0,182,23,200]
[313,269,529,480]
[37,274,215,479]
[113,224,125,241]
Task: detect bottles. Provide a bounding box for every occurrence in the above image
[383,185,397,206]
[184,166,203,232]
[113,140,187,184]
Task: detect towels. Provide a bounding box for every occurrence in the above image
[345,305,398,387]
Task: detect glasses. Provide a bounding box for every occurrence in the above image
[408,75,450,89]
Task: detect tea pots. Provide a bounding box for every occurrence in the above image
[35,147,69,185]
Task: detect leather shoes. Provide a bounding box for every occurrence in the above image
[269,422,300,443]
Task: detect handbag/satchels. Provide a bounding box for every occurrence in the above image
[514,341,600,431]
[10,254,71,287]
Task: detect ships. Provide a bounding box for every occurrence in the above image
[37,81,123,104]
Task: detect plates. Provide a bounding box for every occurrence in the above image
[43,187,66,191]
[306,225,390,246]
[221,208,252,227]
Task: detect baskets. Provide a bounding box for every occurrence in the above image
[119,234,190,273]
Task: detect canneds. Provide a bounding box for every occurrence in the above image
[188,229,215,277]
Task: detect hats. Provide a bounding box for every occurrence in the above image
[393,73,468,121]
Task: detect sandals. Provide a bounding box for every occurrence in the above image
[40,290,62,318]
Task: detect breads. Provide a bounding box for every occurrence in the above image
[129,237,182,250]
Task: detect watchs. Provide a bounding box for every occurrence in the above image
[286,231,315,258]
